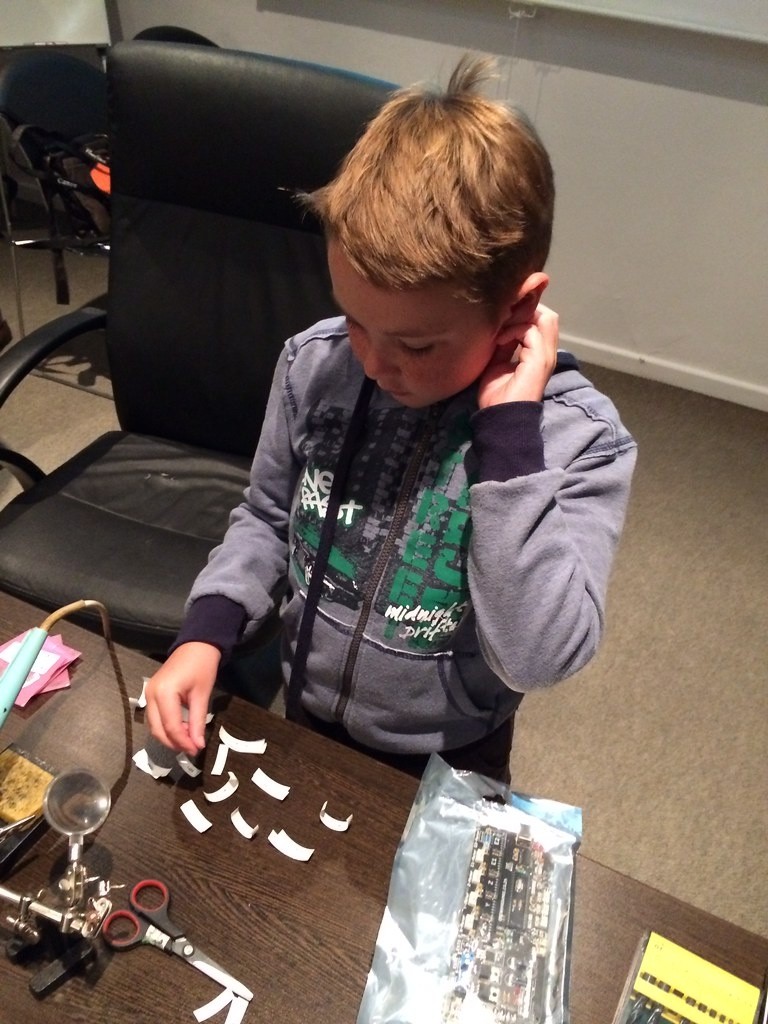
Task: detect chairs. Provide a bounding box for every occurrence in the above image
[0,38,412,711]
[0,25,224,303]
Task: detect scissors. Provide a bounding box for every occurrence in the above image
[102,878,256,1003]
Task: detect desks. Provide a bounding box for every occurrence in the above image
[0,594,768,1024]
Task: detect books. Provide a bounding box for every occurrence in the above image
[610,927,760,1024]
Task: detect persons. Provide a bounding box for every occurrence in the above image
[144,86,640,806]
